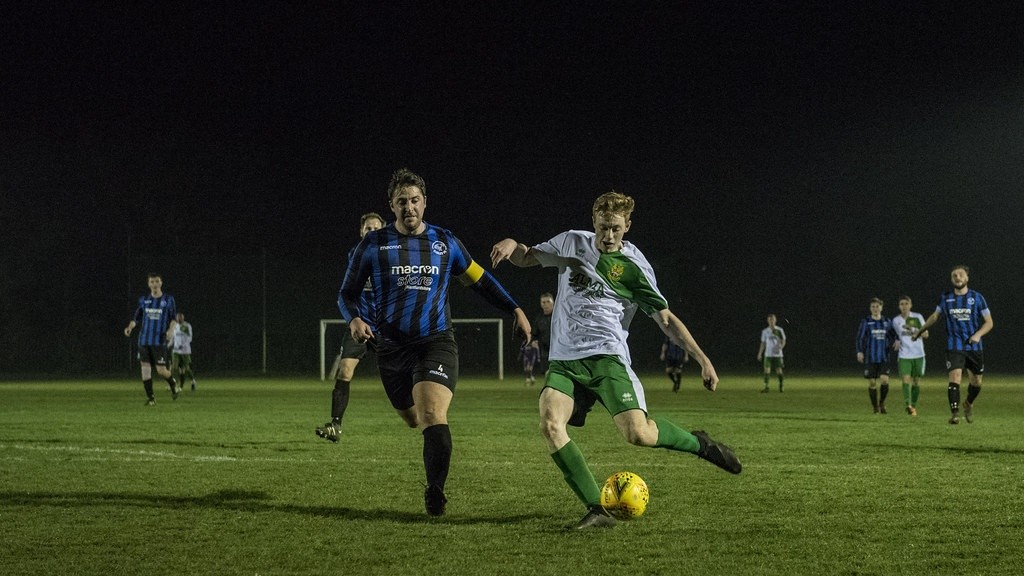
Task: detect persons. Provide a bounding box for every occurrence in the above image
[315,212,386,442]
[892,296,929,415]
[912,265,994,423]
[491,190,742,529]
[855,298,900,414]
[660,336,688,393]
[531,292,554,374]
[757,314,786,393]
[124,273,180,406]
[337,168,532,514]
[516,338,540,386]
[166,313,196,390]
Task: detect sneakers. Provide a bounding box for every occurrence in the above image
[425,486,446,516]
[570,509,610,530]
[316,421,341,442]
[692,430,742,474]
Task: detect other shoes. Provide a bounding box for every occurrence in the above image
[170,381,178,400]
[146,399,155,406]
[963,401,974,423]
[191,382,196,390]
[905,406,916,416]
[874,405,878,413]
[879,404,887,414]
[761,388,769,393]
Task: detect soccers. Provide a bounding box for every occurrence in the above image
[600,472,649,521]
